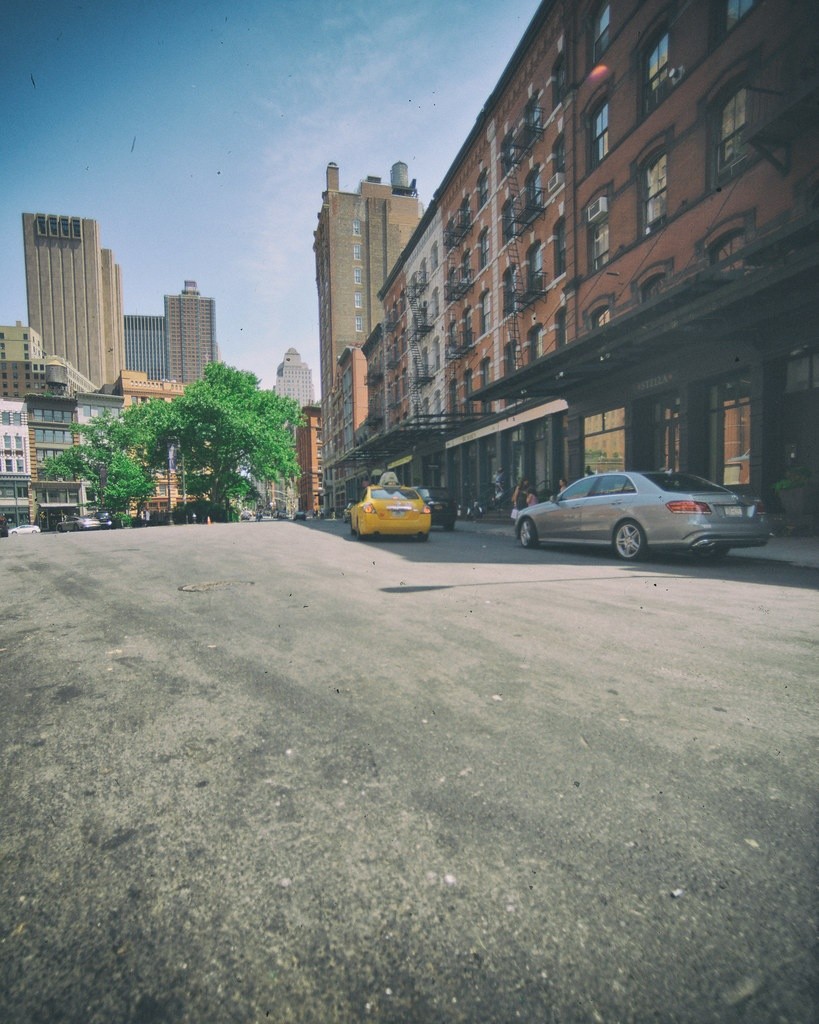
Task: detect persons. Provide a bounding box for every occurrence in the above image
[493,466,595,521]
[255,505,336,521]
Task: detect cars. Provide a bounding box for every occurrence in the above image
[349,472,433,542]
[411,484,457,532]
[93,511,123,529]
[8,524,41,536]
[56,515,102,531]
[342,501,358,523]
[514,469,769,563]
[293,511,306,521]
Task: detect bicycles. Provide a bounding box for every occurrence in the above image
[458,497,483,523]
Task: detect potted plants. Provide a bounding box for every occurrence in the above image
[771,466,819,514]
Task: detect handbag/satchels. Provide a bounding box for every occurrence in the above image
[510,506,519,520]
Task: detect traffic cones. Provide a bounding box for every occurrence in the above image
[206,516,211,524]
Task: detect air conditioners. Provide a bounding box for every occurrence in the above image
[419,300,427,310]
[547,172,564,193]
[587,197,607,222]
